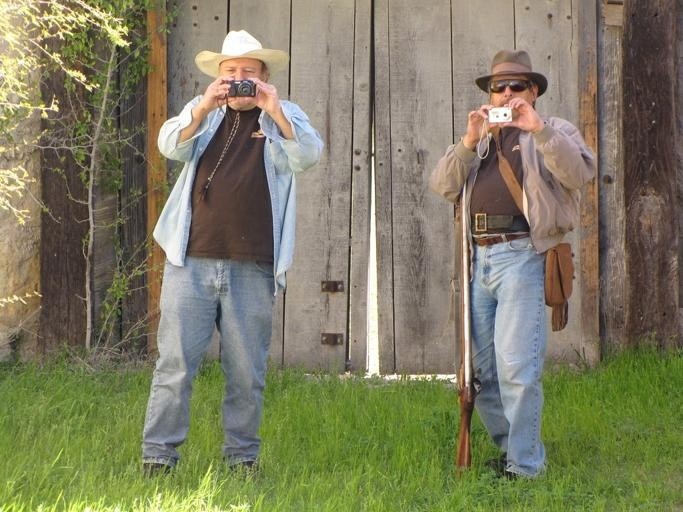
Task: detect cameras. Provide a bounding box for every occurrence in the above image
[220,80,256,97]
[489,107,512,123]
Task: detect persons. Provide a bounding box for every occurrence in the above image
[144,29,325,478]
[428,50,597,482]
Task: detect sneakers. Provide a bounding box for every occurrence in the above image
[485,453,506,475]
[143,463,174,479]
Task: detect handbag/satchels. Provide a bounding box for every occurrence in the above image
[544,243,575,332]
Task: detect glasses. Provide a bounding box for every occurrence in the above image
[484,79,533,93]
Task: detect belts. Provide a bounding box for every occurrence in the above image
[471,212,529,234]
[472,234,530,247]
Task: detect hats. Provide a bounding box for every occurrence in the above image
[194,30,290,78]
[474,49,548,96]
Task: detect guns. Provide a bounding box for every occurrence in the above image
[455,178,476,468]
